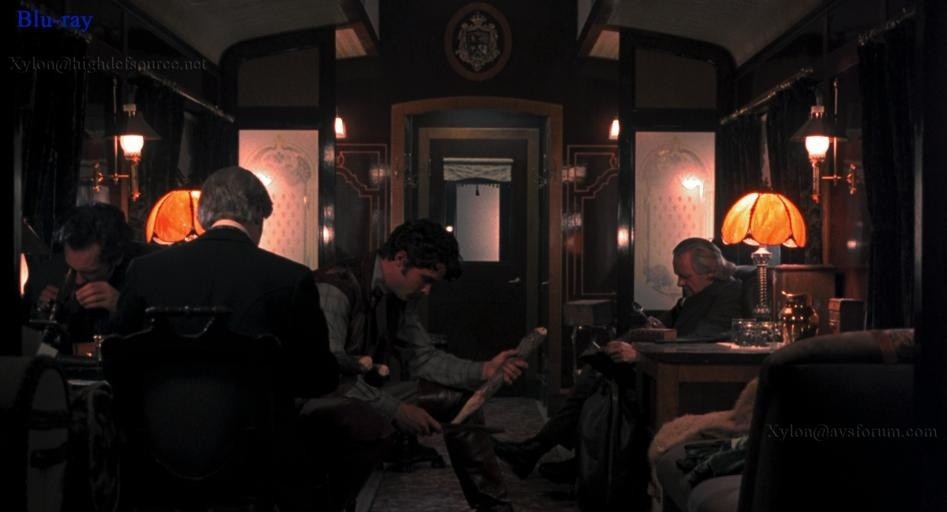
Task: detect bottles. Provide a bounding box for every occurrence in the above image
[778,289,818,344]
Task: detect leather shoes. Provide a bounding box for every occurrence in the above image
[486,436,538,477]
[539,458,581,486]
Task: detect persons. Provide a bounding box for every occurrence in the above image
[490,238,753,484]
[23,201,167,358]
[103,167,340,510]
[294,216,531,510]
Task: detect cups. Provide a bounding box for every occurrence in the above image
[732,321,781,346]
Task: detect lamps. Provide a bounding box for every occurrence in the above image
[86,84,161,204]
[721,191,808,322]
[789,101,858,204]
[146,189,207,248]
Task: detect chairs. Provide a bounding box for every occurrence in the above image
[649,327,915,512]
[97,303,341,511]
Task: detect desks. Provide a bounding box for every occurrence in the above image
[631,340,777,434]
